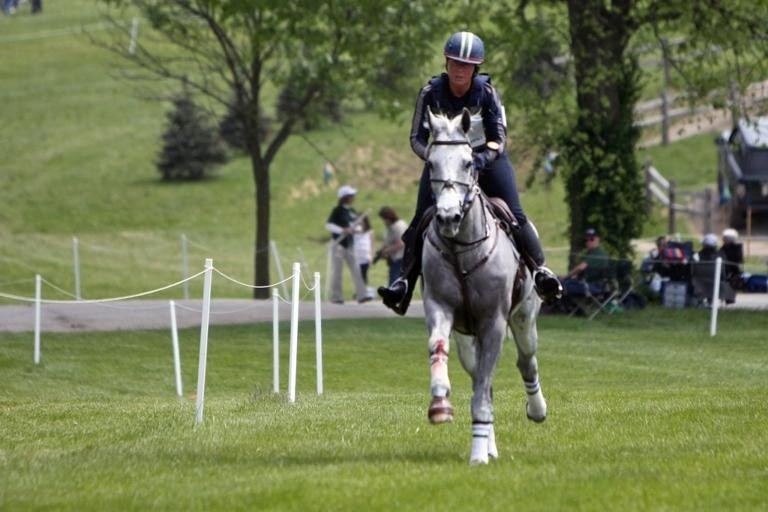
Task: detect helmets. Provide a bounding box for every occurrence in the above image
[444,31,484,65]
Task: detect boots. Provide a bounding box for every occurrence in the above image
[533,267,564,306]
[377,266,414,316]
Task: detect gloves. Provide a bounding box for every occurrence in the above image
[472,150,498,172]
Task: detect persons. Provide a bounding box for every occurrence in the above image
[377,31,565,316]
[638,227,745,309]
[0,0,43,17]
[376,205,410,288]
[548,228,611,316]
[325,185,376,304]
[350,212,375,300]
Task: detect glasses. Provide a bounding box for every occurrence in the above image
[584,238,594,242]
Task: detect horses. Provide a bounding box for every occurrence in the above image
[421,105,547,466]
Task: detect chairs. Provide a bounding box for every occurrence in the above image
[607,261,634,316]
[564,267,619,321]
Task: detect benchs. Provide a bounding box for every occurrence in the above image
[642,260,740,307]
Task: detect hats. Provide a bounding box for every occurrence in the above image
[336,186,357,198]
[584,228,597,239]
